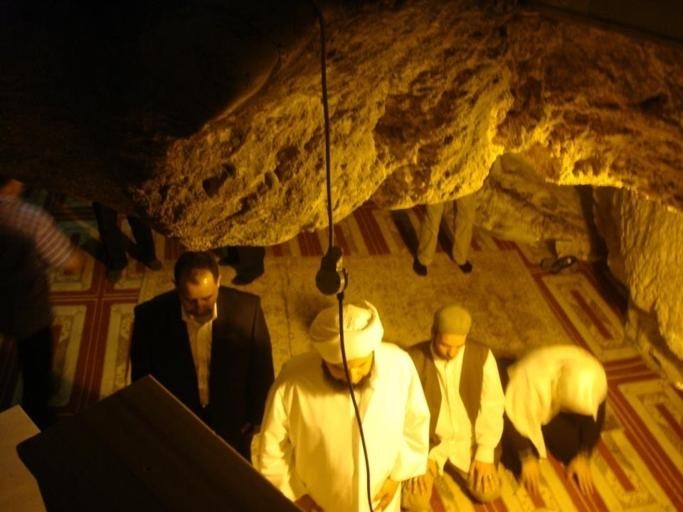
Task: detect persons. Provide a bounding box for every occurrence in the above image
[412,198,473,277]
[502,340,613,499]
[92,198,162,283]
[397,305,510,512]
[0,168,87,431]
[251,297,431,512]
[217,241,266,287]
[127,248,276,467]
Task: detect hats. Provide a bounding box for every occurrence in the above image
[432,303,472,337]
[308,298,385,364]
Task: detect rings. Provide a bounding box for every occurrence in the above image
[488,475,493,480]
[412,477,416,482]
